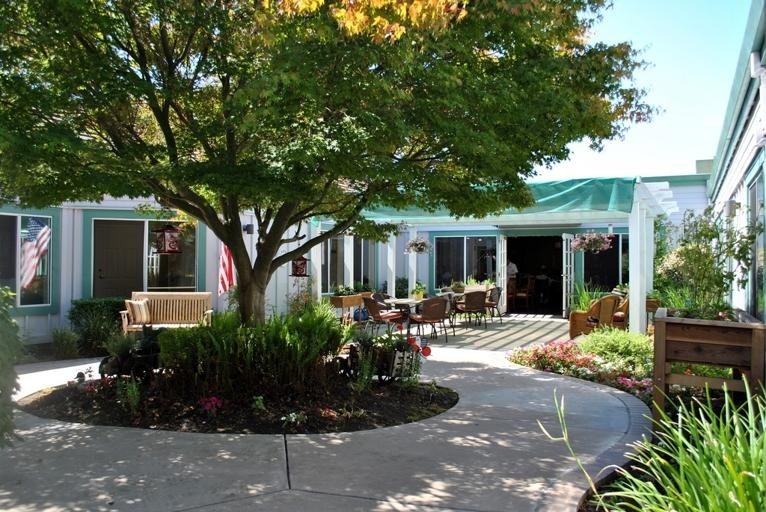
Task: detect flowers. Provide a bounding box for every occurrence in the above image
[404,235,434,257]
[568,233,614,256]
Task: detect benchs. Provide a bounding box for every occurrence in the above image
[116,290,215,341]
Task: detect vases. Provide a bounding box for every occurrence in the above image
[414,244,425,253]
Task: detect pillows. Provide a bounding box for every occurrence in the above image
[123,297,152,326]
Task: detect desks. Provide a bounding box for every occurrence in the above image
[330,294,362,325]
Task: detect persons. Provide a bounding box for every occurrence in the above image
[507,258,518,301]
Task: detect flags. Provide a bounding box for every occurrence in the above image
[18,216,51,291]
[216,242,237,298]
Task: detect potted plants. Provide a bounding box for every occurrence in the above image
[452,282,465,293]
[358,284,372,298]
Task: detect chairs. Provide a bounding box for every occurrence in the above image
[568,292,631,340]
[361,286,506,342]
[508,276,538,313]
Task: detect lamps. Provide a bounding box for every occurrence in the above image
[150,222,184,255]
[288,255,312,278]
